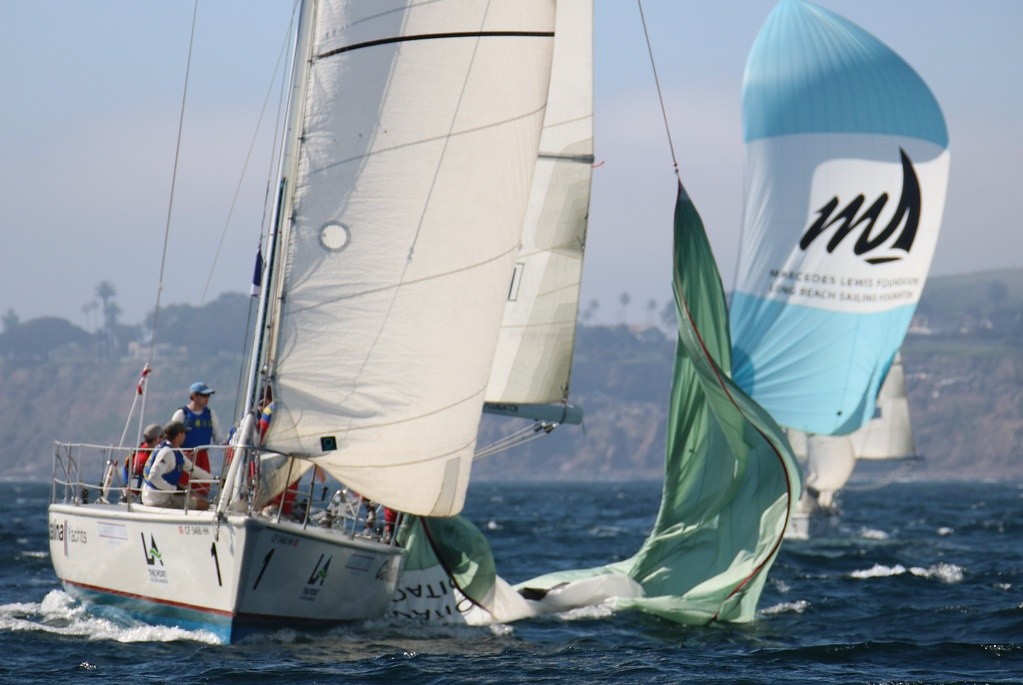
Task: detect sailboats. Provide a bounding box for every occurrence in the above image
[44,0,596,648]
[841,348,927,497]
[781,351,893,562]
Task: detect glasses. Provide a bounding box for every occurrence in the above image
[196,393,211,398]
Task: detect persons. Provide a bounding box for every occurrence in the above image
[127,382,272,511]
[316,486,397,544]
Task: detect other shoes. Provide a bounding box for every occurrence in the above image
[380,531,401,548]
[354,528,376,539]
[262,504,293,523]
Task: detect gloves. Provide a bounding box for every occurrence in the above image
[174,485,196,496]
[213,476,226,489]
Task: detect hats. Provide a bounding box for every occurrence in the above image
[163,420,192,437]
[143,424,165,440]
[189,381,216,394]
